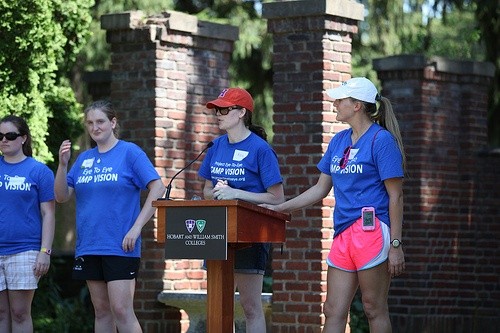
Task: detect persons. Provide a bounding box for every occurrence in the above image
[196,88,284,333]
[256,77,406,333]
[0,114,55,333]
[53,100,167,333]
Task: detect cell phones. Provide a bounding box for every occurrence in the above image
[362,207,375,231]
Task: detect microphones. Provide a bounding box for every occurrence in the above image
[155,141,213,200]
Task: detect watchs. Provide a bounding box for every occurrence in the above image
[39,247,51,255]
[390,238,403,248]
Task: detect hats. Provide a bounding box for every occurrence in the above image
[326,76,378,104]
[206,87,254,114]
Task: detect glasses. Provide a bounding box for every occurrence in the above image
[215,106,242,115]
[0,131,25,141]
